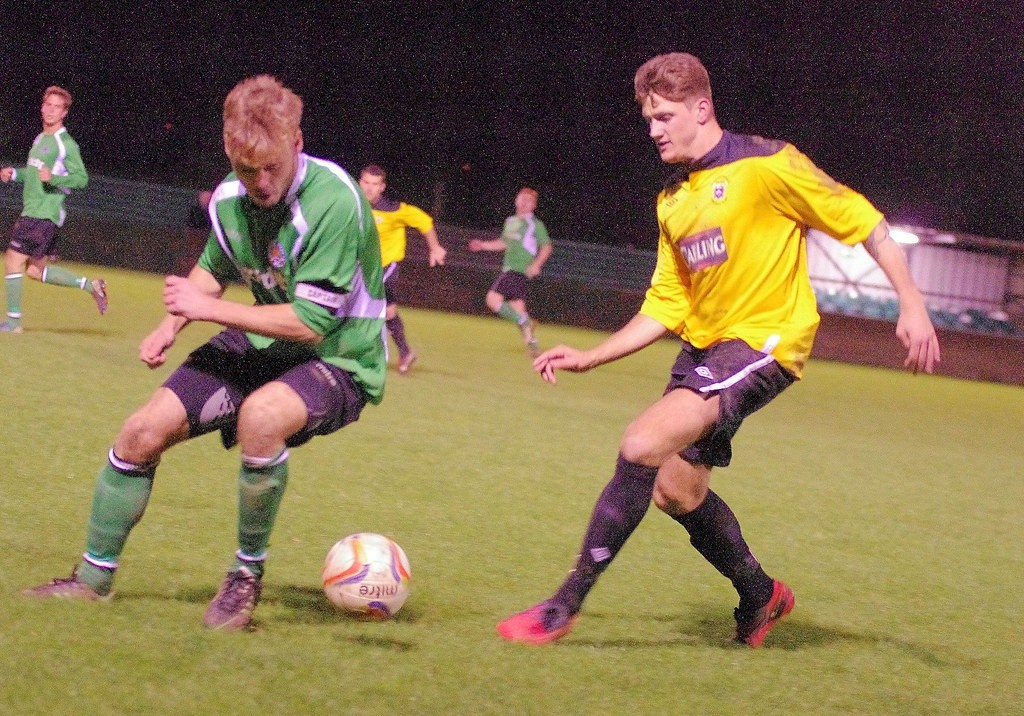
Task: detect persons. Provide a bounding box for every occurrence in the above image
[470,188,552,356]
[359,165,447,374]
[498,53,941,649]
[23,76,389,634]
[0,85,108,334]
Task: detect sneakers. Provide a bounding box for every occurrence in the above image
[203,569,260,631]
[19,577,113,603]
[498,601,573,644]
[0,323,23,335]
[90,278,108,316]
[730,579,795,647]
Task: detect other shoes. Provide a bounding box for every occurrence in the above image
[523,316,533,344]
[400,351,416,373]
[526,341,543,359]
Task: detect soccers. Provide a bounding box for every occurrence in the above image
[320,532,413,620]
